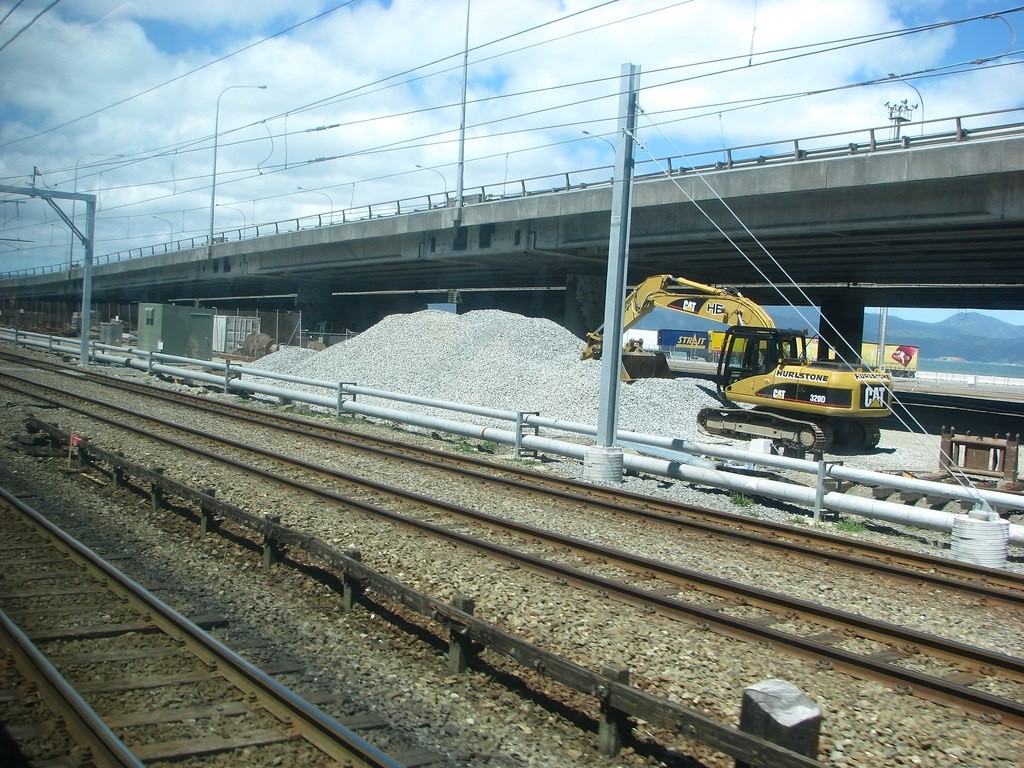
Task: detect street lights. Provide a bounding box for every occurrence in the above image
[296,186,334,225]
[208,84,268,245]
[152,216,173,253]
[51,222,70,270]
[887,71,925,137]
[416,164,449,205]
[69,150,125,269]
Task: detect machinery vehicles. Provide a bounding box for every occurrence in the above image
[694,322,897,454]
[581,273,790,384]
